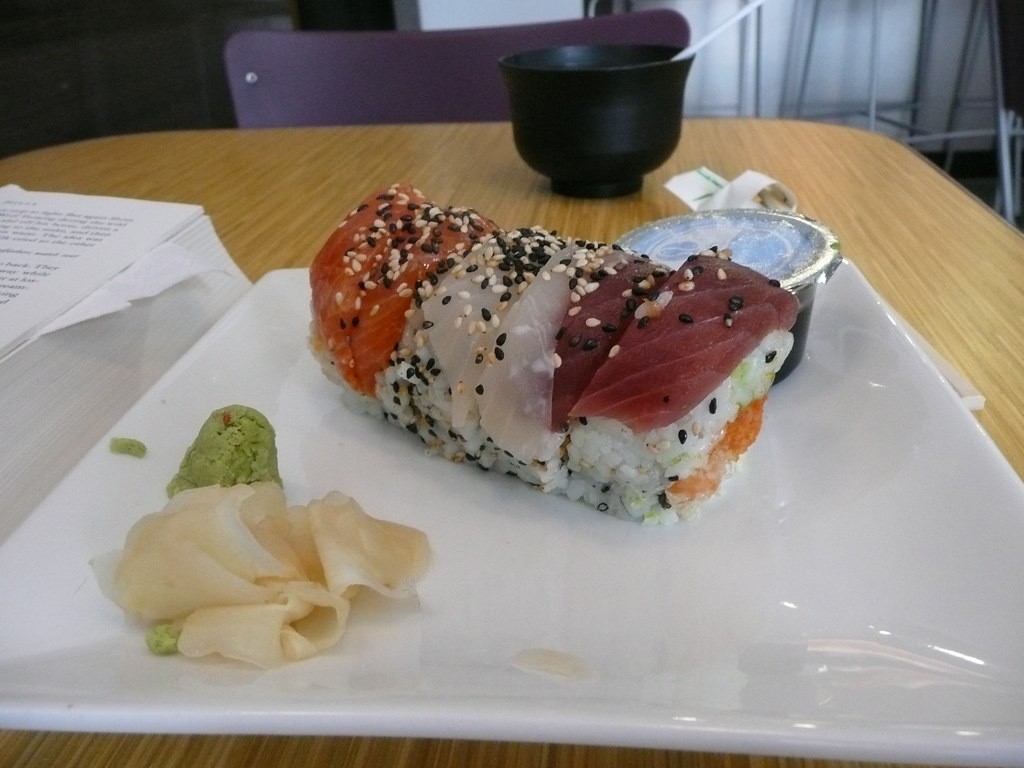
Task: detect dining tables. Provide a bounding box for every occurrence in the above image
[0,115,1023,768]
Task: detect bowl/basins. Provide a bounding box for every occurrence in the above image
[614,208,841,384]
[496,43,696,199]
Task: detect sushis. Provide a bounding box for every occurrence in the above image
[315,181,807,528]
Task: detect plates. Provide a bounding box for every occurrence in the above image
[1,258,1023,768]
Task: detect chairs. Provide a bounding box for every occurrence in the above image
[224,7,691,128]
